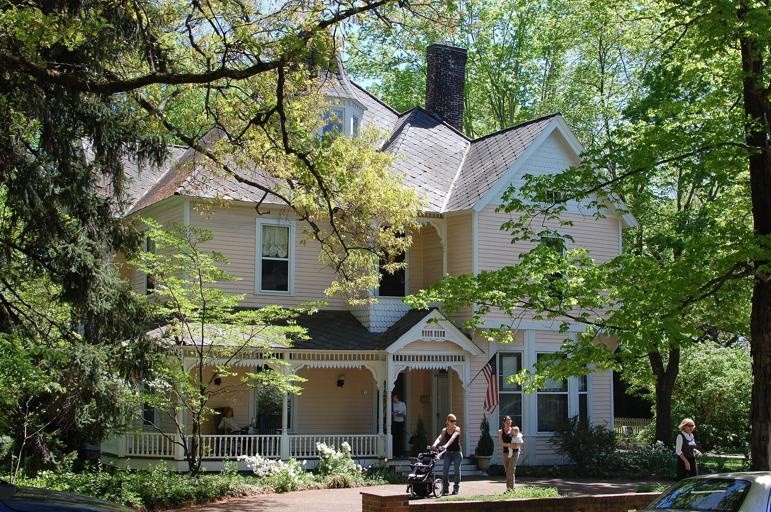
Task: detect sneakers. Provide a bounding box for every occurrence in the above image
[441,489,460,496]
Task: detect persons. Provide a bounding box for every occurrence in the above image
[431,413,464,496]
[217,406,243,435]
[392,392,408,459]
[675,417,704,482]
[507,425,525,460]
[497,415,522,495]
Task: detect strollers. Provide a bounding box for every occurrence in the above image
[406,446,446,498]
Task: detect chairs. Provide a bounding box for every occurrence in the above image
[212,406,253,449]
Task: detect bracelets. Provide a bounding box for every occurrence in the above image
[515,443,518,446]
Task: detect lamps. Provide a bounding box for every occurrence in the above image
[336,374,347,390]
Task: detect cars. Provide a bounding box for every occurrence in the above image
[643,471,771,512]
[0,479,139,512]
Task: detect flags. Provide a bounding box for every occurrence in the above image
[481,352,500,415]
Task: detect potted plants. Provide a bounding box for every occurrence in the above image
[408,414,494,469]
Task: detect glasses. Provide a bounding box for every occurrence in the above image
[448,419,457,422]
[686,424,694,427]
